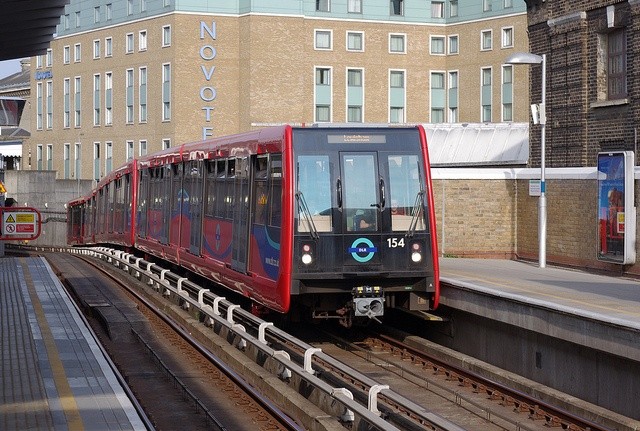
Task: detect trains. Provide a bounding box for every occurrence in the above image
[67,124,439,336]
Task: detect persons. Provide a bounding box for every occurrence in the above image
[355,209,376,232]
[390,197,401,215]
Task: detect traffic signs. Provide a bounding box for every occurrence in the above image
[529,178,541,197]
[0,206,41,241]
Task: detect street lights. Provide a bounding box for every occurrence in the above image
[509,53,547,270]
